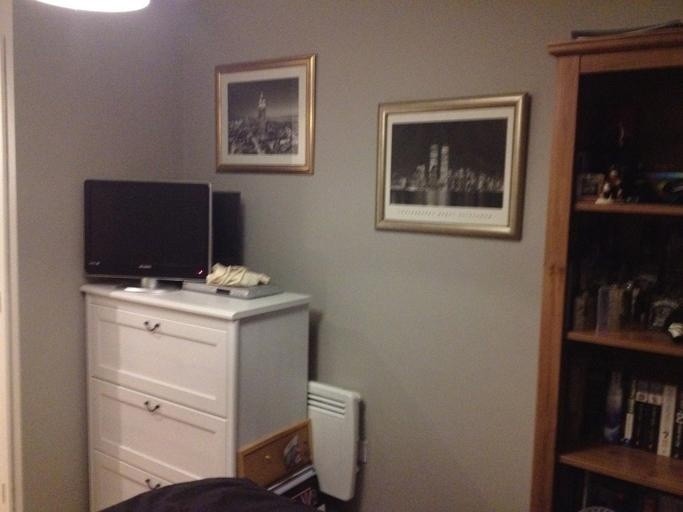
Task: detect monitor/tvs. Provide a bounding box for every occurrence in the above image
[82,178,214,295]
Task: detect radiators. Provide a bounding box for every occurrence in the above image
[307,379,364,500]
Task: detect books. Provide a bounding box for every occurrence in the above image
[563,258,682,512]
[265,464,315,492]
[270,469,326,511]
[569,18,682,40]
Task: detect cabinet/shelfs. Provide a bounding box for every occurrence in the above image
[78,281,311,512]
[529,25,682,512]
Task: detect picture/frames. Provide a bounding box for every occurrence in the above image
[267,466,322,509]
[376,91,530,241]
[237,419,311,490]
[214,55,315,175]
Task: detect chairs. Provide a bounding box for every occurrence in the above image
[87,477,322,512]
